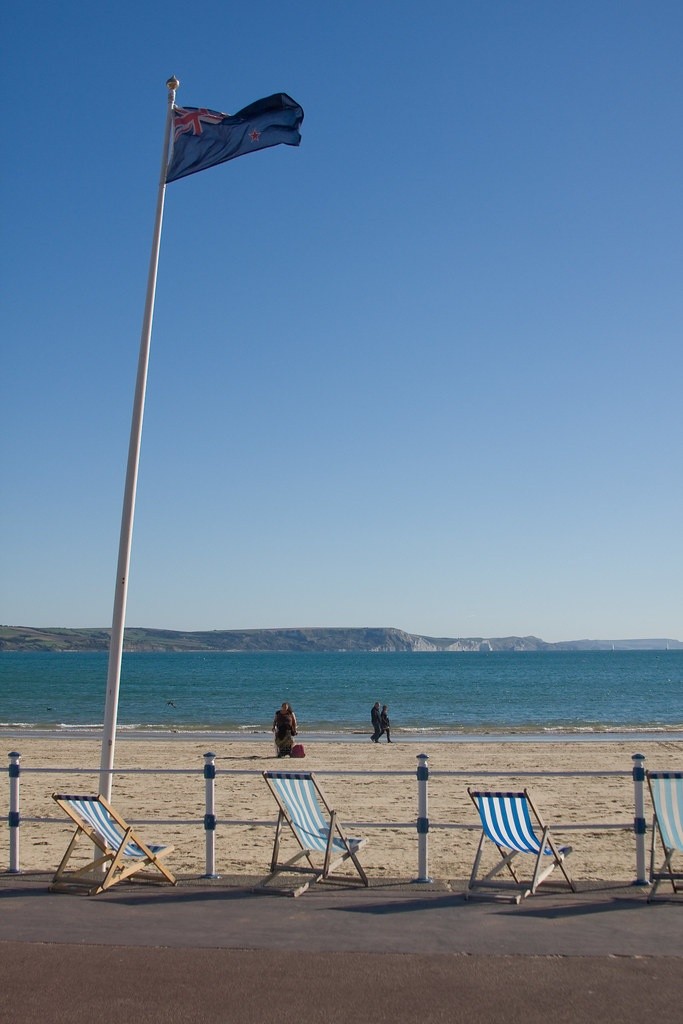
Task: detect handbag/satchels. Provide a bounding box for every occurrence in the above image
[292,744,305,758]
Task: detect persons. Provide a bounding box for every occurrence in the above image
[376,704,392,743]
[272,702,297,758]
[369,702,383,743]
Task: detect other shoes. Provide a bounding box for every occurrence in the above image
[371,737,374,742]
[388,741,393,743]
[277,752,291,758]
[375,740,378,743]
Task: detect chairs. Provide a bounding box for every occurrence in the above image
[261,770,369,897]
[48,792,179,897]
[645,770,683,905]
[466,786,580,904]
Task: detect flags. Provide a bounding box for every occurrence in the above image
[165,92,305,187]
